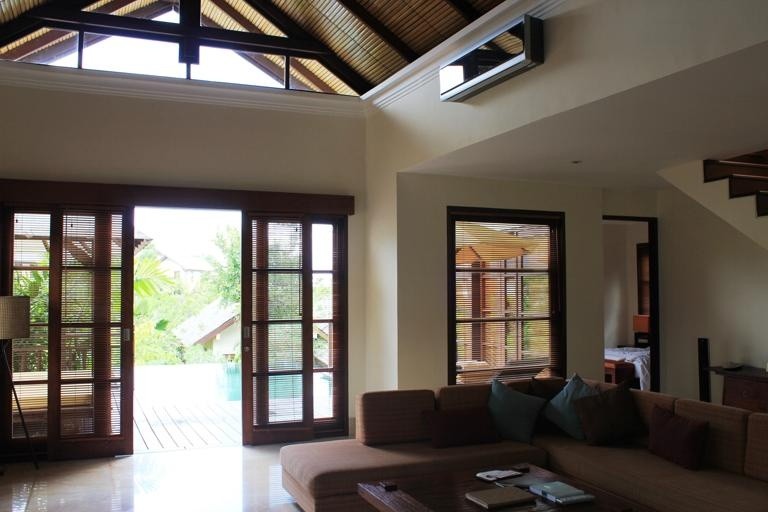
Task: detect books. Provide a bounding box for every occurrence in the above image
[530,482,584,502]
[465,486,536,509]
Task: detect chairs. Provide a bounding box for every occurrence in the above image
[458,356,549,384]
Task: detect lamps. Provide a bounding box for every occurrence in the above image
[1,294,46,472]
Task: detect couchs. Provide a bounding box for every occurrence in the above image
[276,377,768,510]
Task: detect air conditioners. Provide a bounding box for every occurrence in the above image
[439,14,546,103]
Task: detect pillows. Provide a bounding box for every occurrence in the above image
[571,377,641,449]
[546,370,599,442]
[421,401,502,451]
[488,378,550,449]
[657,405,712,473]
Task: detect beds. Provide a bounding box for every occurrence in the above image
[603,331,649,392]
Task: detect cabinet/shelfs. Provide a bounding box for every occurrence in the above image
[709,363,768,414]
